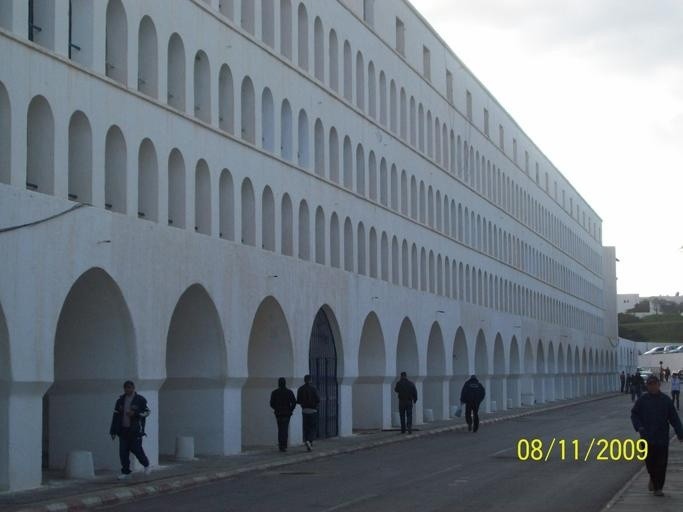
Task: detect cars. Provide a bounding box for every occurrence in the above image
[639,371,653,388]
[644,344,683,354]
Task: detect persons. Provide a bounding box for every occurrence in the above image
[670,372,681,412]
[108,378,154,483]
[630,374,682,497]
[664,366,670,383]
[268,375,296,453]
[297,373,320,453]
[619,370,645,403]
[392,371,417,436]
[659,360,666,382]
[458,374,486,434]
[105,382,106,383]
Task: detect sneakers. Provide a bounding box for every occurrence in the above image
[143,462,151,475]
[116,473,131,479]
[648,479,665,496]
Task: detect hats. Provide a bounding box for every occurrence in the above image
[645,374,659,385]
